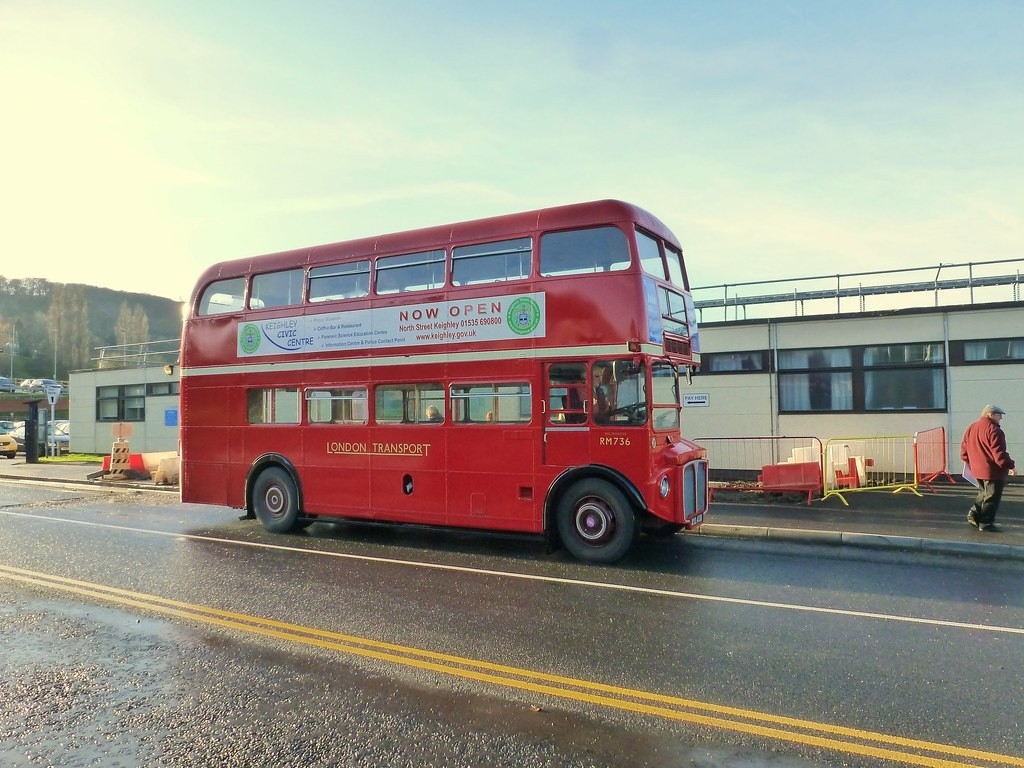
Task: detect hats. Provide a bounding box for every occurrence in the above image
[983,405,1005,414]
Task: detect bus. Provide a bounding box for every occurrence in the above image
[180,198,710,564]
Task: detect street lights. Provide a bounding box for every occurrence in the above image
[121,329,126,367]
[53,329,56,379]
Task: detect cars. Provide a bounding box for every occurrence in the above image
[29,378,64,393]
[0,419,69,436]
[0,426,17,459]
[21,379,34,391]
[0,376,15,392]
[10,425,69,457]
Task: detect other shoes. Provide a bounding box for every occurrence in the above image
[979,523,1002,532]
[967,510,980,527]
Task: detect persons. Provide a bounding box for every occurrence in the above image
[565,366,609,423]
[426,405,444,423]
[961,405,1017,532]
